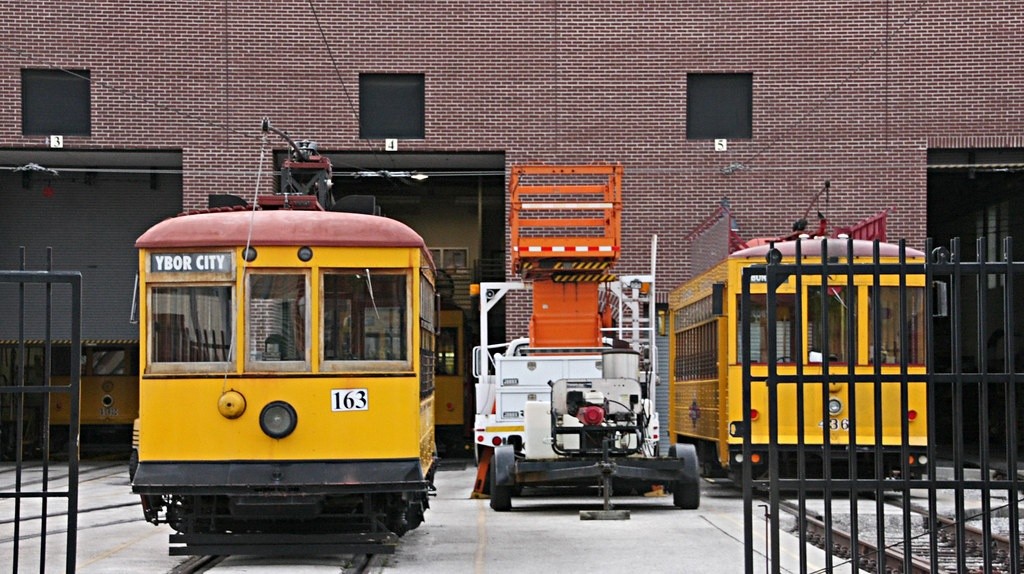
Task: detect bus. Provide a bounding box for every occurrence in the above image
[0,309,473,458]
[130,114,443,558]
[657,179,928,492]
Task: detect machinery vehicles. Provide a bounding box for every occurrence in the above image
[471,162,703,524]
